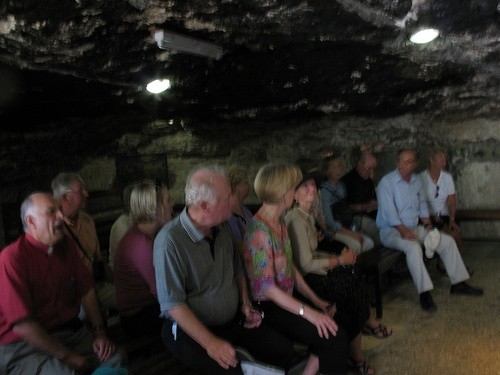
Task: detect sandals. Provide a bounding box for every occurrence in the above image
[347,355,376,375]
[362,322,394,339]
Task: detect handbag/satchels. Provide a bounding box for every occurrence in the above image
[331,197,354,228]
[93,258,107,282]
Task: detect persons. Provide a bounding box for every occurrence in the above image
[340,150,378,226]
[0,192,129,375]
[418,147,462,271]
[151,164,297,375]
[376,149,484,312]
[113,178,175,332]
[109,179,140,272]
[243,160,363,375]
[226,164,254,250]
[287,170,394,340]
[50,171,119,310]
[319,156,395,257]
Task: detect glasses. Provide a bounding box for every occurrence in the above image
[435,186,439,198]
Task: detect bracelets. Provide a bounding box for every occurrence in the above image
[242,299,252,306]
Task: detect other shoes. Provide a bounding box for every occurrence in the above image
[451,281,484,296]
[419,292,437,314]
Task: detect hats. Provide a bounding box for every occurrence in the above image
[424,228,441,258]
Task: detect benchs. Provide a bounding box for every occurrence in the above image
[0,207,500,375]
